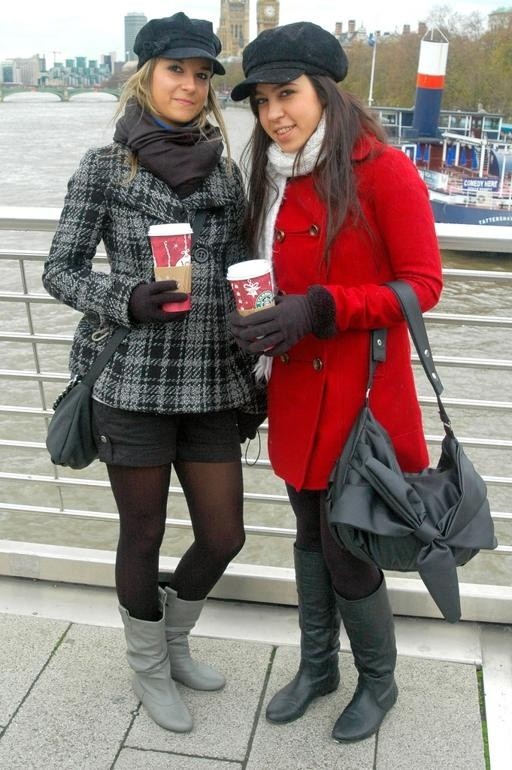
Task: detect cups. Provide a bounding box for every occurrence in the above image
[226,259,277,353]
[148,223,194,313]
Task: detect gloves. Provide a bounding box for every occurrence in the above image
[228,284,336,360]
[127,281,188,322]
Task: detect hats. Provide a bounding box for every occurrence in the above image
[231,20,348,100]
[134,12,226,79]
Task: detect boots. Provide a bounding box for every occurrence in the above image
[330,570,399,744]
[117,587,193,734]
[265,540,341,726]
[163,581,226,693]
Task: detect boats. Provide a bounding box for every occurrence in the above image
[342,13,511,255]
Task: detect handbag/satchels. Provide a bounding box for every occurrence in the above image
[45,384,99,470]
[326,276,503,573]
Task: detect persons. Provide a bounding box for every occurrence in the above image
[230,22,443,744]
[41,11,252,732]
